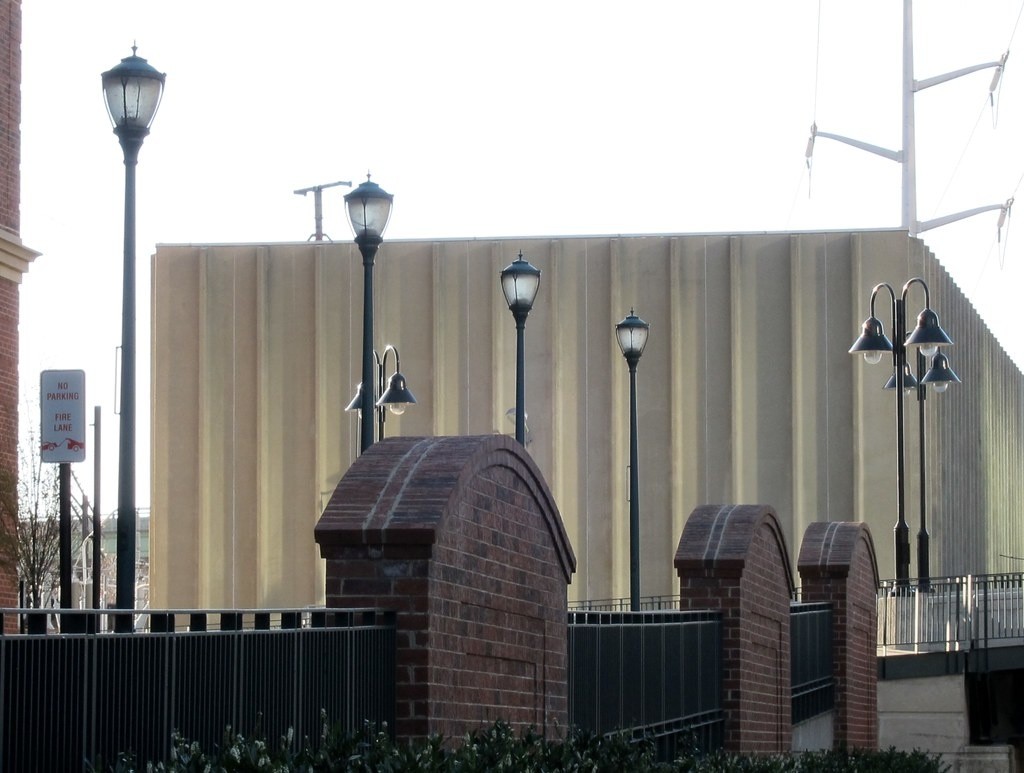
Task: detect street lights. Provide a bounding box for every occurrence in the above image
[339,170,397,450]
[847,277,953,596]
[345,344,416,452]
[611,308,659,609]
[881,328,961,602]
[498,251,541,445]
[97,42,169,632]
